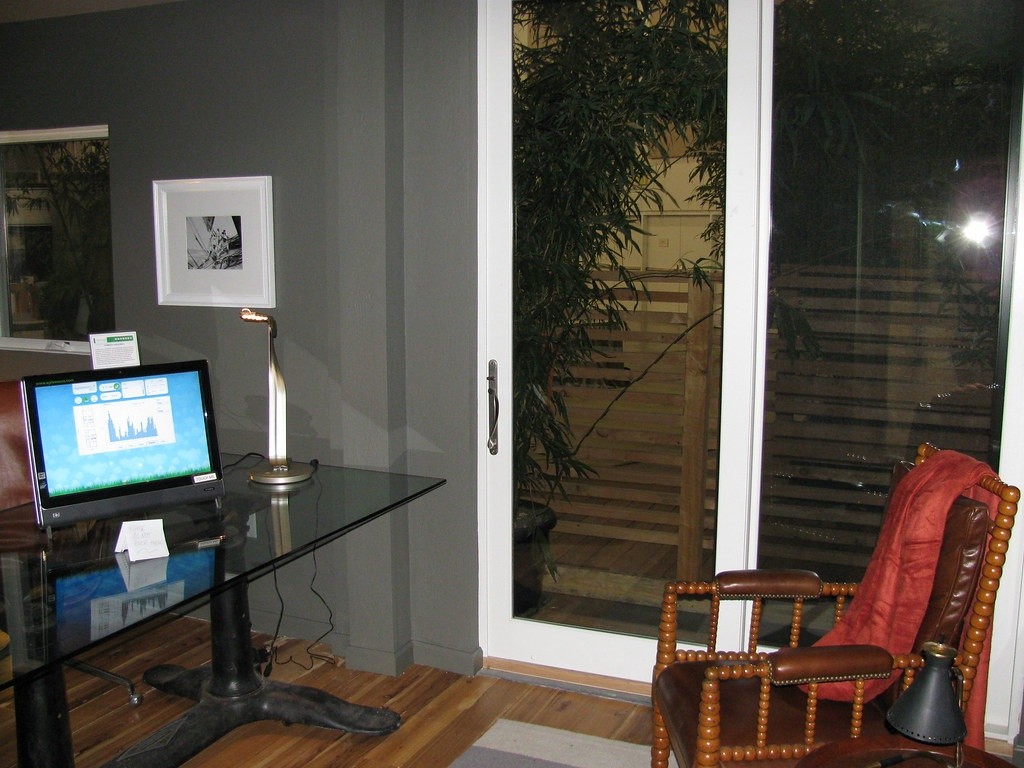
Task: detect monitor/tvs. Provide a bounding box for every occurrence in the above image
[47,534,226,658]
[19,358,226,530]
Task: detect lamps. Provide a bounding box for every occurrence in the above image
[886,641,968,768]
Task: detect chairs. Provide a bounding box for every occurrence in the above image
[652,444,1019,768]
[0,381,144,708]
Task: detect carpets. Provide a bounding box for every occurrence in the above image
[446,718,679,768]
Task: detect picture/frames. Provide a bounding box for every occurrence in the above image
[153,174,275,308]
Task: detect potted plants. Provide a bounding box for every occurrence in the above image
[512,1,1018,618]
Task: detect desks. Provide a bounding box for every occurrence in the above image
[0,454,448,768]
